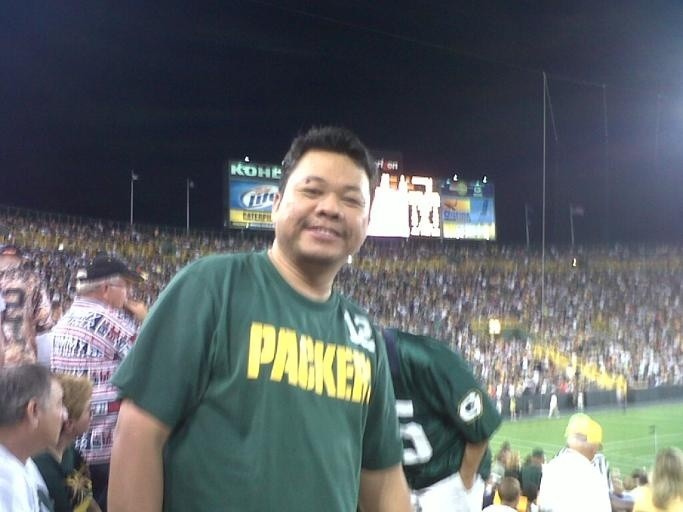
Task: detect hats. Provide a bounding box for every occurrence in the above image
[76,257,144,282]
[564,414,602,444]
